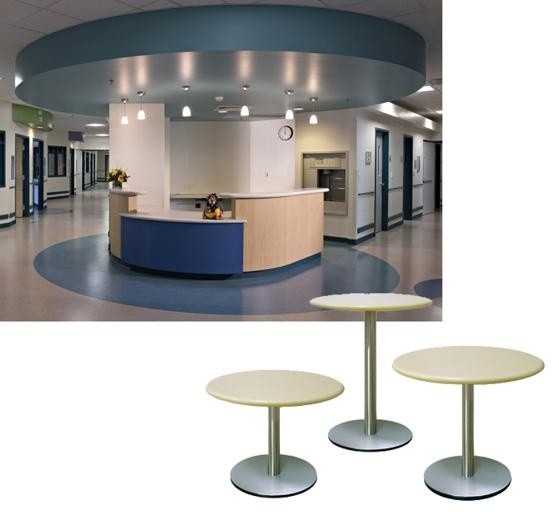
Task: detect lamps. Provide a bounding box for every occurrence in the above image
[240,85,250,117]
[136,91,146,120]
[284,90,295,120]
[181,85,192,118]
[309,97,319,124]
[121,98,129,125]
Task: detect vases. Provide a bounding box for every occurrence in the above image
[111,184,122,191]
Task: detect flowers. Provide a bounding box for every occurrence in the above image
[105,166,131,188]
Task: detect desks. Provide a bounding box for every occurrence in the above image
[393,346,544,500]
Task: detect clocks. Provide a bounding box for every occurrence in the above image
[278,125,293,140]
